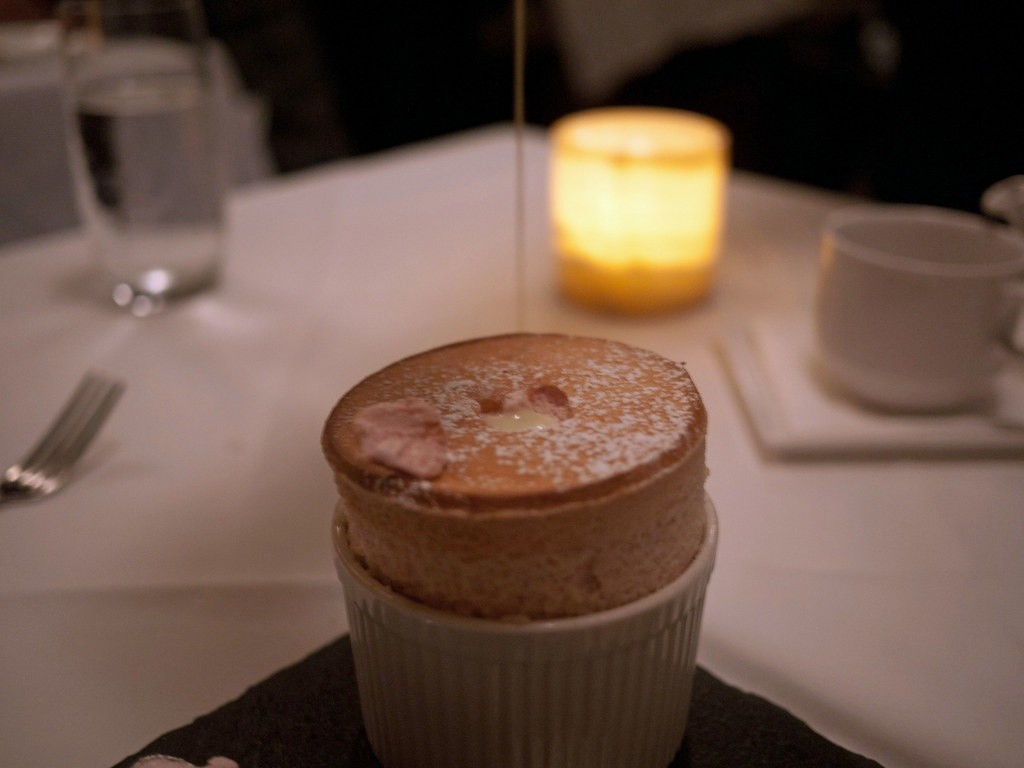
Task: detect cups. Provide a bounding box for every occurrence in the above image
[50,1,230,306]
[813,210,1024,417]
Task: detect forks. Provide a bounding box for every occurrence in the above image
[0,369,127,505]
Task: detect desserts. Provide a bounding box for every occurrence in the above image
[317,329,710,622]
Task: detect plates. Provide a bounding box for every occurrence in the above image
[715,312,1024,453]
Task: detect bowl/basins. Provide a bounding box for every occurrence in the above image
[332,496,717,767]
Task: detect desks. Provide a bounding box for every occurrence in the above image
[0,121,1024,768]
